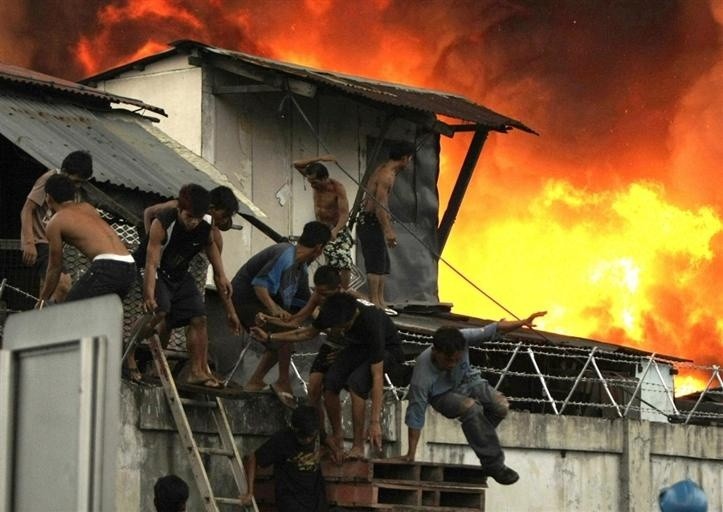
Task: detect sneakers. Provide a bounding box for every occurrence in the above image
[489,465,518,485]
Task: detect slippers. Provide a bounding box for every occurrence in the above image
[243,384,271,393]
[189,375,224,390]
[269,382,299,411]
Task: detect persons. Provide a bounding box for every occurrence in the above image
[19,150,93,304]
[292,154,353,290]
[357,142,415,314]
[240,406,349,512]
[389,310,547,485]
[154,476,190,512]
[124,185,246,391]
[229,222,404,462]
[34,175,137,309]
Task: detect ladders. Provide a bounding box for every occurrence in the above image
[148,328,260,512]
